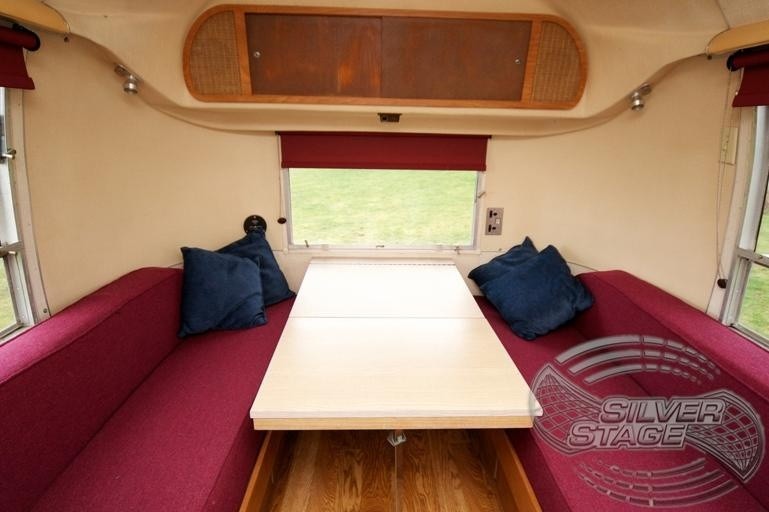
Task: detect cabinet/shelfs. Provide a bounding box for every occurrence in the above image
[182,1,589,111]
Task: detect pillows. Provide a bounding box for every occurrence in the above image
[179,246,266,337]
[216,232,295,308]
[469,238,538,288]
[487,247,593,339]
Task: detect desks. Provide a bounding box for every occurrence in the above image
[250,261,543,477]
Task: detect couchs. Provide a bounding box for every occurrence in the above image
[0,267,295,512]
[474,270,769,512]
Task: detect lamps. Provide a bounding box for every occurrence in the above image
[125,76,136,91]
[633,93,643,110]
